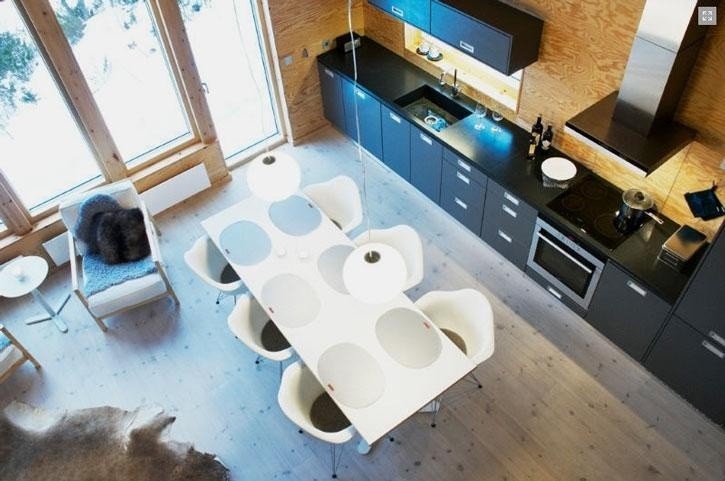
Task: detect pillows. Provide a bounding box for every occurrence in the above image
[59,180,150,266]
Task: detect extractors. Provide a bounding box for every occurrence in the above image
[563,0,721,179]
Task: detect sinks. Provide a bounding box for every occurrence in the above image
[393,84,474,134]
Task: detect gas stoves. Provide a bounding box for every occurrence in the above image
[546,173,658,251]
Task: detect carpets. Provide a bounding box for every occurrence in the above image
[0,400,231,481]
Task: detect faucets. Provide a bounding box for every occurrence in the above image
[451,69,462,97]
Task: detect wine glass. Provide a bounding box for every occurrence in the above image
[490,106,506,133]
[473,102,488,131]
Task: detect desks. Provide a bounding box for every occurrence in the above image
[0,256,71,332]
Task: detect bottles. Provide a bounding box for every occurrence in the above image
[529,113,544,149]
[541,123,554,154]
[526,135,537,161]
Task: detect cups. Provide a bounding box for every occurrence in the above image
[419,41,431,53]
[429,47,440,58]
[273,244,312,265]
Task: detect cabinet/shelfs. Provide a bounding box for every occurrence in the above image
[381,103,443,209]
[318,62,383,162]
[441,144,538,271]
[586,219,725,428]
[368,0,544,76]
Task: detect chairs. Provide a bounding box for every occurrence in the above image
[58,178,179,332]
[0,324,41,385]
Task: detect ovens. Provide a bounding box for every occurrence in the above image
[526,211,609,311]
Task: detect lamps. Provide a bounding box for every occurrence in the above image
[233,0,301,200]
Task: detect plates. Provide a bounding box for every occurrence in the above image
[317,244,357,295]
[416,47,429,56]
[218,219,271,267]
[374,305,444,372]
[541,156,578,181]
[317,341,386,409]
[427,52,444,61]
[260,271,321,328]
[267,193,322,237]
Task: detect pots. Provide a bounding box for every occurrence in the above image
[621,188,664,226]
